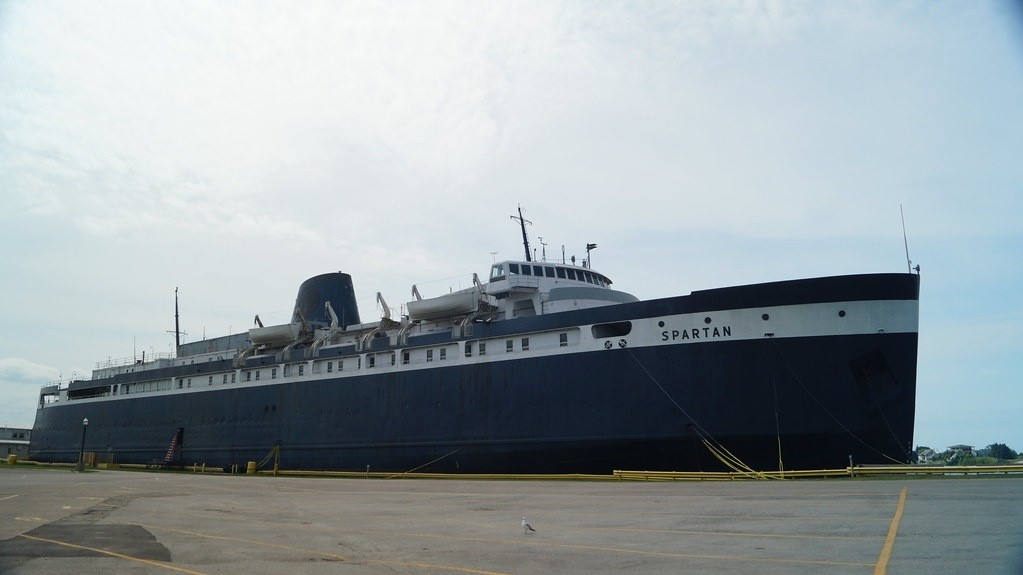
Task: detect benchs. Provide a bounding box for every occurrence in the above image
[145,461,168,470]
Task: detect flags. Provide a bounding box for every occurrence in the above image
[588,243,598,250]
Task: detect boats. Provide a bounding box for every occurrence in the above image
[24,202,924,480]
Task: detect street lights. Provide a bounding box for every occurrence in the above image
[79,417,88,462]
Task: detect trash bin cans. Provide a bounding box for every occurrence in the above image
[246,461,256,474]
[8,455,17,465]
